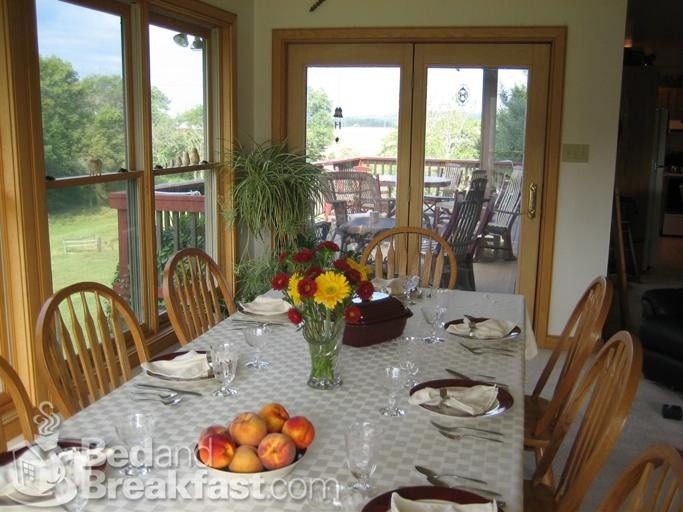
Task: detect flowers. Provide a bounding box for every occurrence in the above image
[273,241,374,379]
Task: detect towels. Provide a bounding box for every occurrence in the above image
[408,384,499,416]
[447,318,518,339]
[239,295,282,313]
[389,492,498,512]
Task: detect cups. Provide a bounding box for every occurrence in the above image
[370,210,379,223]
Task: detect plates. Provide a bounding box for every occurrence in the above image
[360,486,504,512]
[7,435,106,471]
[410,379,513,419]
[237,300,293,315]
[3,473,78,507]
[444,318,521,342]
[145,351,216,383]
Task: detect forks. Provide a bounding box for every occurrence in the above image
[459,343,515,358]
[126,382,201,406]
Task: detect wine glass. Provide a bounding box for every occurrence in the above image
[380,338,424,417]
[117,413,152,475]
[421,286,450,344]
[400,273,420,306]
[345,421,379,494]
[55,447,90,512]
[243,323,273,369]
[210,343,239,396]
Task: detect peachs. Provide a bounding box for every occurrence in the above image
[227,412,267,447]
[229,444,264,472]
[282,415,315,449]
[198,426,229,444]
[258,433,296,470]
[256,404,286,432]
[197,434,236,469]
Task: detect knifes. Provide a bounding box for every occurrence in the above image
[446,369,509,391]
[464,315,474,320]
[224,320,284,330]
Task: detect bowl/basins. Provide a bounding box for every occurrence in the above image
[193,437,308,483]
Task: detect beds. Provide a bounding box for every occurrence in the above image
[0,288,538,512]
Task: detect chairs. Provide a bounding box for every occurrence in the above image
[323,168,519,289]
[525,276,683,512]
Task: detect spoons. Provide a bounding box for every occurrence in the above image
[430,421,505,444]
[416,466,506,506]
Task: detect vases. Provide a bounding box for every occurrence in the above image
[303,323,344,390]
